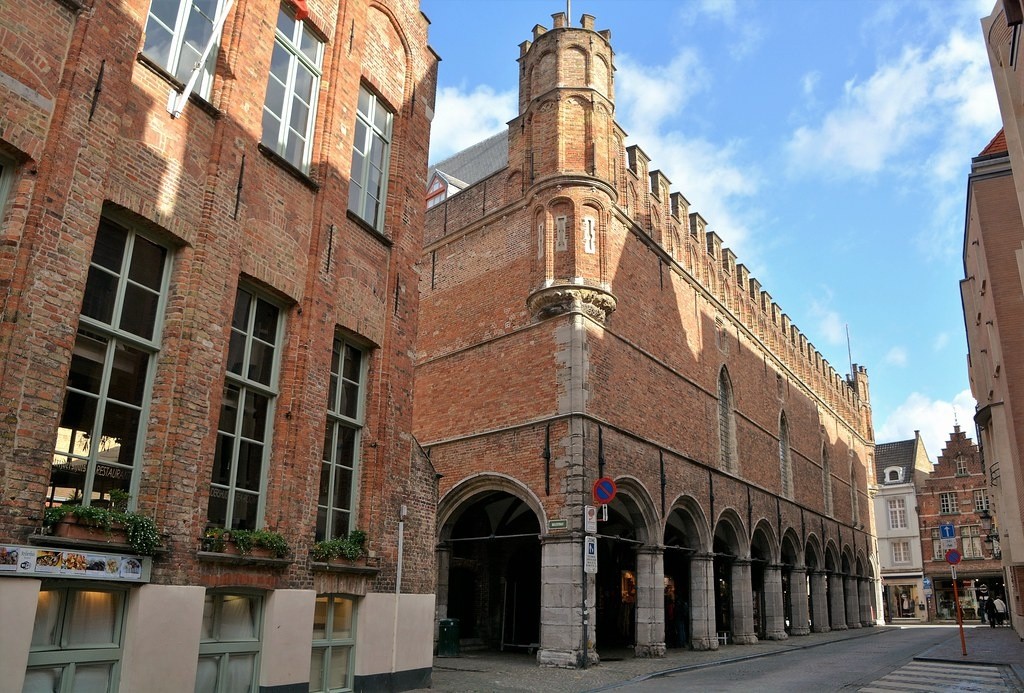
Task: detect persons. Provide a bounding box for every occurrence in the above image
[956,602,965,624]
[993,596,1006,628]
[985,598,998,629]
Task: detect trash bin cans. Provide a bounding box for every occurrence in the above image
[437,618,462,658]
